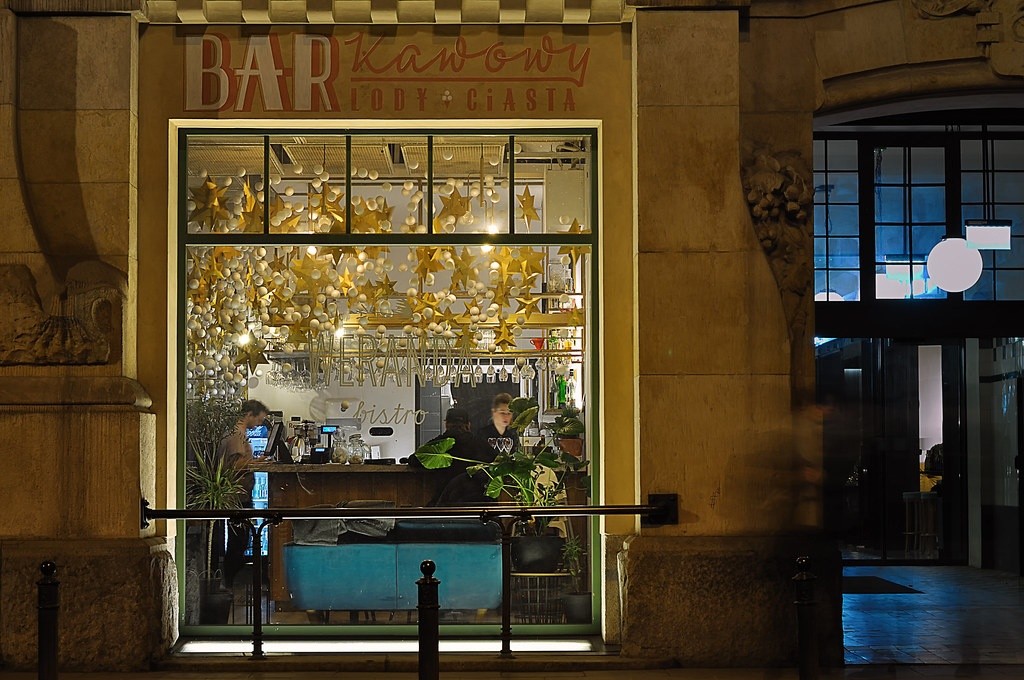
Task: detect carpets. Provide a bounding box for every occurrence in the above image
[842,575,927,595]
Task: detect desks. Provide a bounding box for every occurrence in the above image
[509,573,571,623]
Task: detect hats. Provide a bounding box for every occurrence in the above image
[444,407,472,424]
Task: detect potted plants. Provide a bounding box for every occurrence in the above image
[415,395,590,571]
[556,406,583,456]
[184,433,260,625]
[562,533,591,624]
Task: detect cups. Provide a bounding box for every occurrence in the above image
[530,338,543,349]
[488,437,513,454]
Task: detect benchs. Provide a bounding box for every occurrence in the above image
[282,521,502,623]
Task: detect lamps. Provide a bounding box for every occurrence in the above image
[927,114,983,293]
[964,123,1013,250]
[814,185,846,301]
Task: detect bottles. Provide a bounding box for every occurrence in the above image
[550,369,577,409]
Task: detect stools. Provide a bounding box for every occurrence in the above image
[921,491,943,557]
[903,492,923,553]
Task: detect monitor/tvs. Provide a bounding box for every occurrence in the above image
[264,422,294,464]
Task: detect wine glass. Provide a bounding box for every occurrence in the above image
[265,358,384,393]
[386,355,544,384]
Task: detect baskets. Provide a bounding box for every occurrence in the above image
[198,568,222,593]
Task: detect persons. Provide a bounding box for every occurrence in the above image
[218,399,268,582]
[408,409,508,507]
[477,393,520,459]
